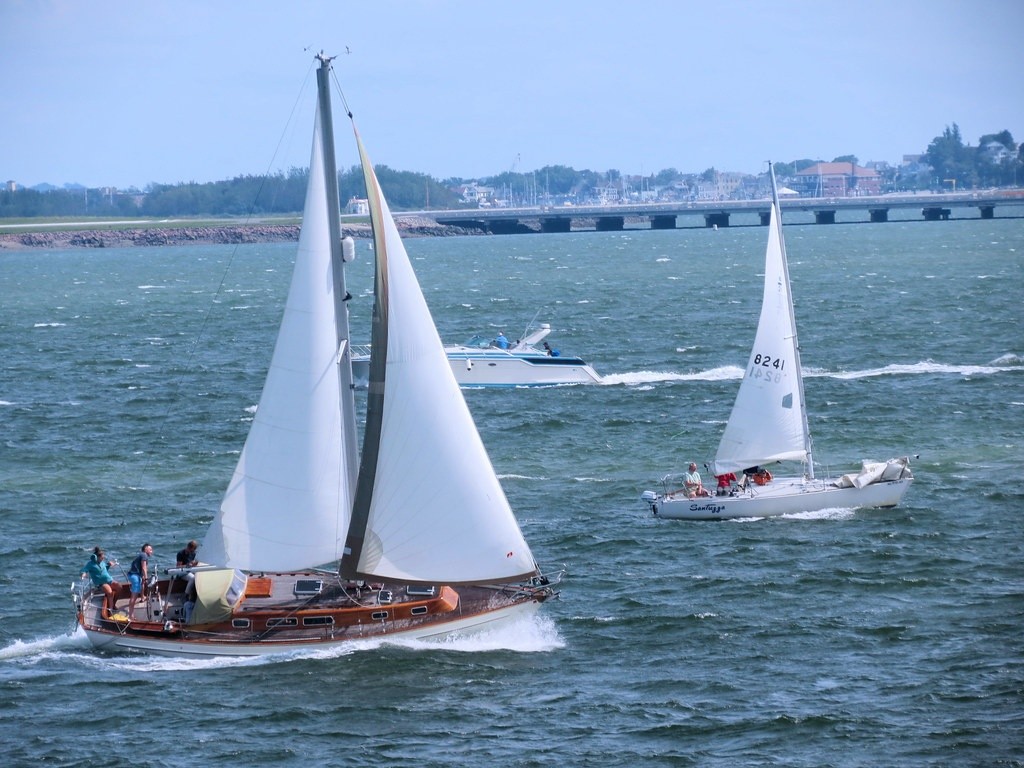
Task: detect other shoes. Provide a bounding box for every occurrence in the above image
[184,593,196,603]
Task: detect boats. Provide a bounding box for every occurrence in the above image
[350,307,604,385]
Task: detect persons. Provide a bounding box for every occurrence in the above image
[80,546,122,617]
[177,541,197,601]
[743,466,772,486]
[714,472,737,496]
[543,342,552,356]
[493,332,507,349]
[683,462,708,498]
[128,544,152,621]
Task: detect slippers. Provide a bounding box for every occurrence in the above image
[140,596,148,603]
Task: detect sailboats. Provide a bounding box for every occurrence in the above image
[71,44,566,659]
[641,160,915,519]
